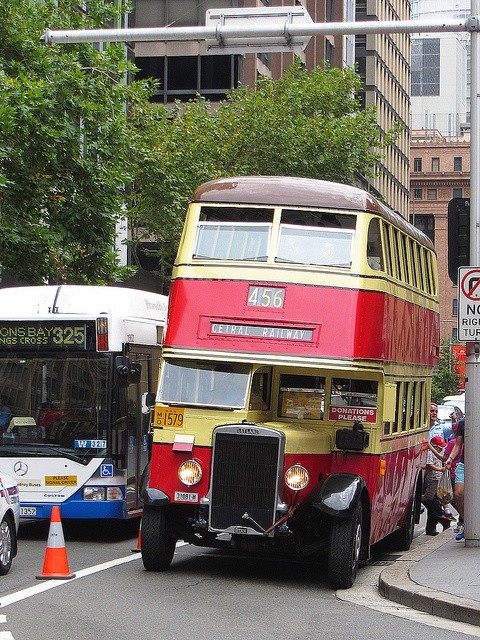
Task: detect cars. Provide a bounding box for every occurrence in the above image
[434,406,464,446]
[0,469,24,574]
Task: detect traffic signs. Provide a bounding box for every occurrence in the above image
[457,263,480,342]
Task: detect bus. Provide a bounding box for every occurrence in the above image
[0,284,170,519]
[140,175,442,589]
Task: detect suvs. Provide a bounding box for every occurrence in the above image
[441,395,465,415]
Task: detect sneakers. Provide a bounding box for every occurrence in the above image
[454,524,460,532]
[442,511,456,520]
[456,525,464,540]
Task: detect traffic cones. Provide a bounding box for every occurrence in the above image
[131,517,142,552]
[35,505,76,580]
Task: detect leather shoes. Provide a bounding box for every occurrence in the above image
[427,529,439,535]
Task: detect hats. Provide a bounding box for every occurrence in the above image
[431,436,447,448]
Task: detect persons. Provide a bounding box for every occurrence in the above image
[422,403,464,541]
